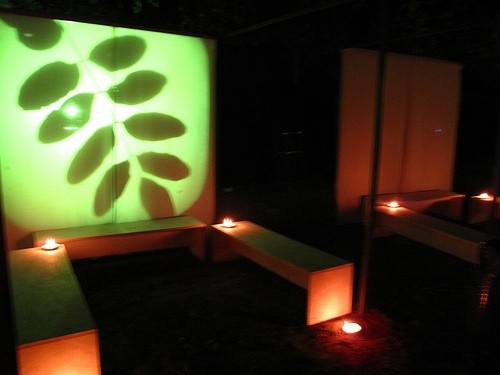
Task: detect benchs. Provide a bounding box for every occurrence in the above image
[33,216,209,262]
[210,221,355,326]
[467,196,495,225]
[373,189,466,236]
[7,243,102,375]
[374,204,495,265]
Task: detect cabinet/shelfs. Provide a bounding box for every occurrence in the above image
[334,48,463,224]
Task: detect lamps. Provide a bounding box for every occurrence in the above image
[342,321,362,333]
[222,218,236,227]
[42,238,59,249]
[386,201,400,207]
[476,192,489,198]
